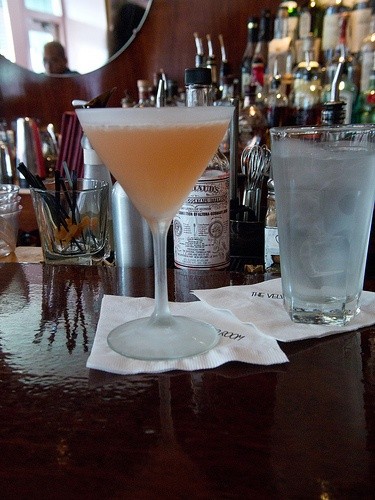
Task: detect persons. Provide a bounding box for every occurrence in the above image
[38,41,81,77]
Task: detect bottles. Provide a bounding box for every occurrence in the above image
[71,0,375,271]
[16,117,48,188]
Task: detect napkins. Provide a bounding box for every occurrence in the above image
[190,278,375,342]
[84,294,291,375]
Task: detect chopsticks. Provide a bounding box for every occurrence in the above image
[17,162,100,254]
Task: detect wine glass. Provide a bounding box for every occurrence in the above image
[76,106,236,361]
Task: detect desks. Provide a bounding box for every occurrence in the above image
[0,259,375,500]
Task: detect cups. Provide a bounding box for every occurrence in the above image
[29,179,110,263]
[37,260,110,329]
[0,184,23,256]
[0,252,30,316]
[269,123,375,327]
